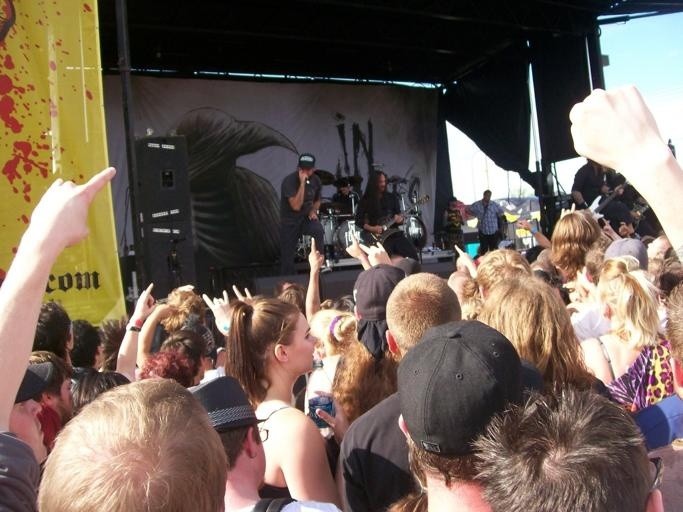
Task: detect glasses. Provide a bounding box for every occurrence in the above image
[647,455,664,494]
[256,425,268,443]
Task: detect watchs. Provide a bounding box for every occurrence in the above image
[124,324,141,334]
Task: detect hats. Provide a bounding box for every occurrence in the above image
[185,375,269,433]
[14,361,54,405]
[603,239,647,271]
[396,319,544,457]
[178,324,218,365]
[352,263,410,322]
[297,153,316,169]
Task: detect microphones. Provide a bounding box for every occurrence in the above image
[306,178,311,185]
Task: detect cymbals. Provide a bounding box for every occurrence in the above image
[329,202,346,208]
[320,202,330,211]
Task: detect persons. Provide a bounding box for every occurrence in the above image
[572,85,683,269]
[334,265,407,439]
[464,190,505,251]
[329,178,360,223]
[226,295,341,511]
[573,155,607,214]
[279,152,333,274]
[115,283,195,388]
[27,350,75,423]
[136,304,205,382]
[5,364,54,470]
[300,237,354,322]
[69,318,105,373]
[389,322,545,512]
[345,230,421,280]
[582,255,682,511]
[442,196,466,259]
[0,167,227,512]
[72,373,130,408]
[472,386,666,512]
[337,273,461,512]
[357,171,420,262]
[616,194,641,221]
[476,276,617,403]
[33,301,73,366]
[550,208,605,286]
[186,378,344,511]
[157,289,204,334]
[452,237,533,322]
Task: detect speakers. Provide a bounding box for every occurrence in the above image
[128,136,199,301]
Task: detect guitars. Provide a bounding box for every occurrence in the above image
[357,194,431,249]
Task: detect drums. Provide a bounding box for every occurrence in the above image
[317,211,339,245]
[333,219,373,257]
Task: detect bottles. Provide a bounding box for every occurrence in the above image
[307,360,337,438]
